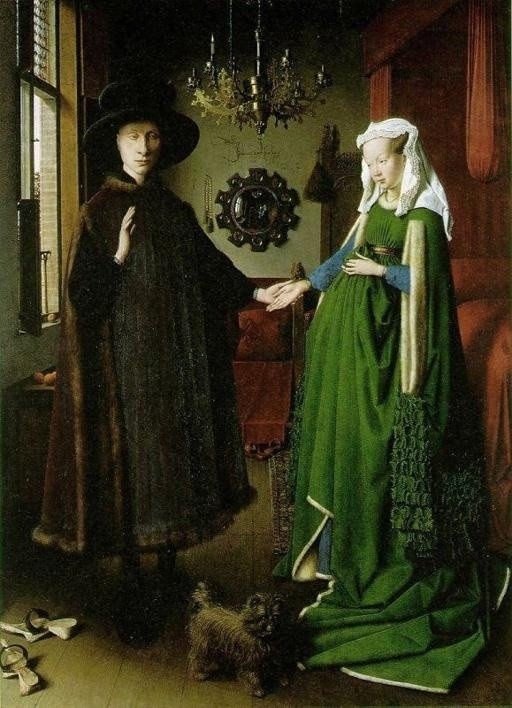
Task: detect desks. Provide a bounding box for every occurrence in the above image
[5,383,64,544]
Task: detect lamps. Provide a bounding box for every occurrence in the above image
[185,0,332,134]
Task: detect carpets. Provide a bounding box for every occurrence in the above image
[268,450,296,561]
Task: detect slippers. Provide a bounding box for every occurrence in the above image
[0,607,77,697]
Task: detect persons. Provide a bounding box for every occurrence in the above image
[265,117,512,694]
[31,75,305,648]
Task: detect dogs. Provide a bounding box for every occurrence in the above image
[184,579,291,699]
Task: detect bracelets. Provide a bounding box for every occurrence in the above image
[380,263,386,278]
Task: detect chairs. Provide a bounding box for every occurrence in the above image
[214,263,306,446]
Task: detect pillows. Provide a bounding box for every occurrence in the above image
[235,307,292,361]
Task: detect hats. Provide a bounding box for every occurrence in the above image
[82,80,200,172]
